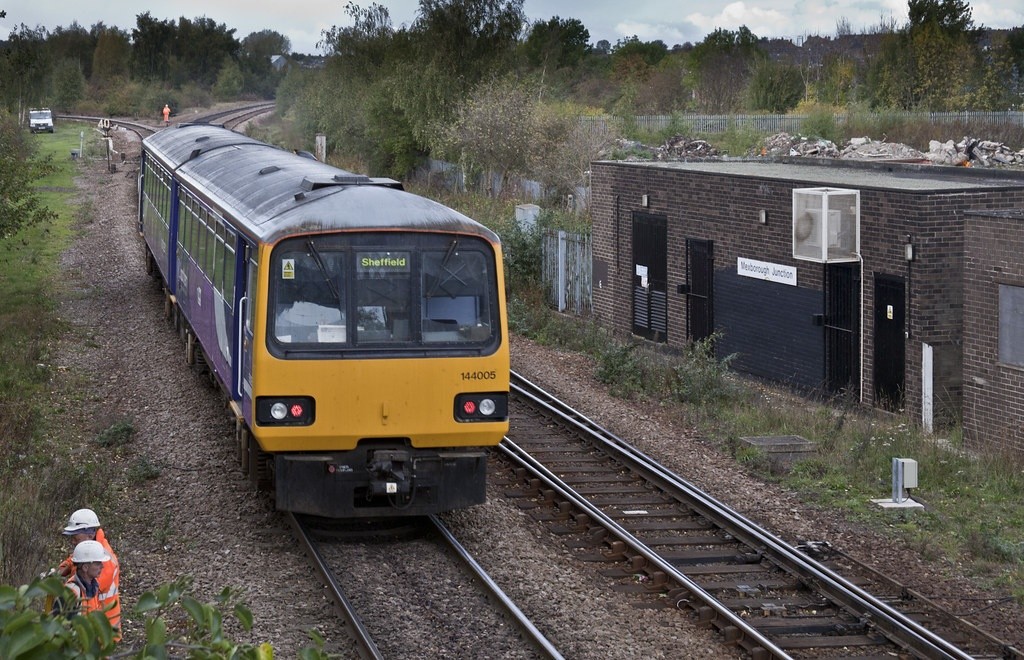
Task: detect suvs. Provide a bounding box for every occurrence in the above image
[29,108,53,133]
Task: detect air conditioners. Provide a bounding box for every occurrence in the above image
[800,208,841,248]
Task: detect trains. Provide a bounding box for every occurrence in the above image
[138,122,509,519]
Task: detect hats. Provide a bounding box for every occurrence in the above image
[62,528,95,535]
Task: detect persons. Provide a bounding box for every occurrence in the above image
[39,508,122,660]
[163,104,171,121]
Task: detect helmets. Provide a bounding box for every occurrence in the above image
[71,540,110,563]
[64,508,101,531]
